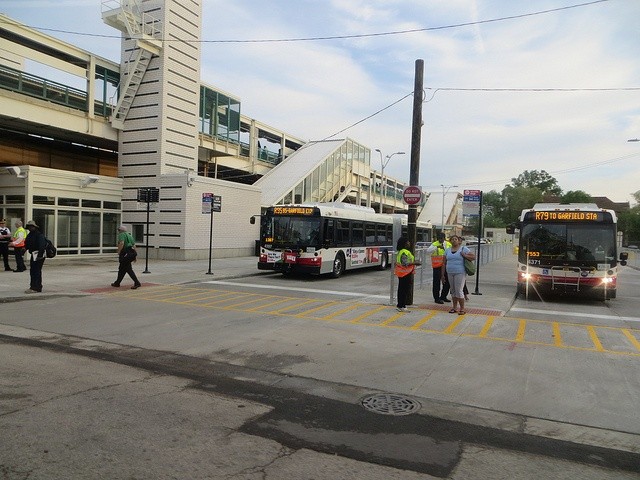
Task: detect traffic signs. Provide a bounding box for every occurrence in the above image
[213,195,221,212]
[202,198,212,203]
[202,193,213,198]
[201,206,212,213]
[463,196,480,202]
[202,202,212,207]
[463,190,481,197]
[462,202,480,215]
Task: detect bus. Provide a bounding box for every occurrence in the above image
[506,203,628,301]
[249,202,434,278]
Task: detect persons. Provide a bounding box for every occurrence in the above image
[0,219,14,271]
[441,235,475,315]
[24,221,47,294]
[110,226,141,289]
[394,237,422,312]
[427,233,452,304]
[8,221,27,272]
[450,236,470,301]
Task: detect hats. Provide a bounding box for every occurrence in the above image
[25,221,39,229]
[117,227,126,232]
[0,219,6,223]
[438,233,445,239]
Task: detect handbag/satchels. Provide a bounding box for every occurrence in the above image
[45,238,57,258]
[464,259,475,276]
[119,246,137,264]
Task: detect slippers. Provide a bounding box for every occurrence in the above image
[449,309,458,313]
[459,310,466,315]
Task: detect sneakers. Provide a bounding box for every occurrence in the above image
[23,288,39,293]
[396,307,410,313]
[465,296,469,301]
[440,296,451,302]
[111,283,120,287]
[13,269,23,272]
[23,267,26,271]
[405,304,417,309]
[131,283,141,289]
[5,268,14,271]
[435,299,444,304]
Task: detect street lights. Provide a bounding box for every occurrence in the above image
[375,148,406,214]
[440,184,460,234]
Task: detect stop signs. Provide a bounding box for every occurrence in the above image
[402,186,423,205]
